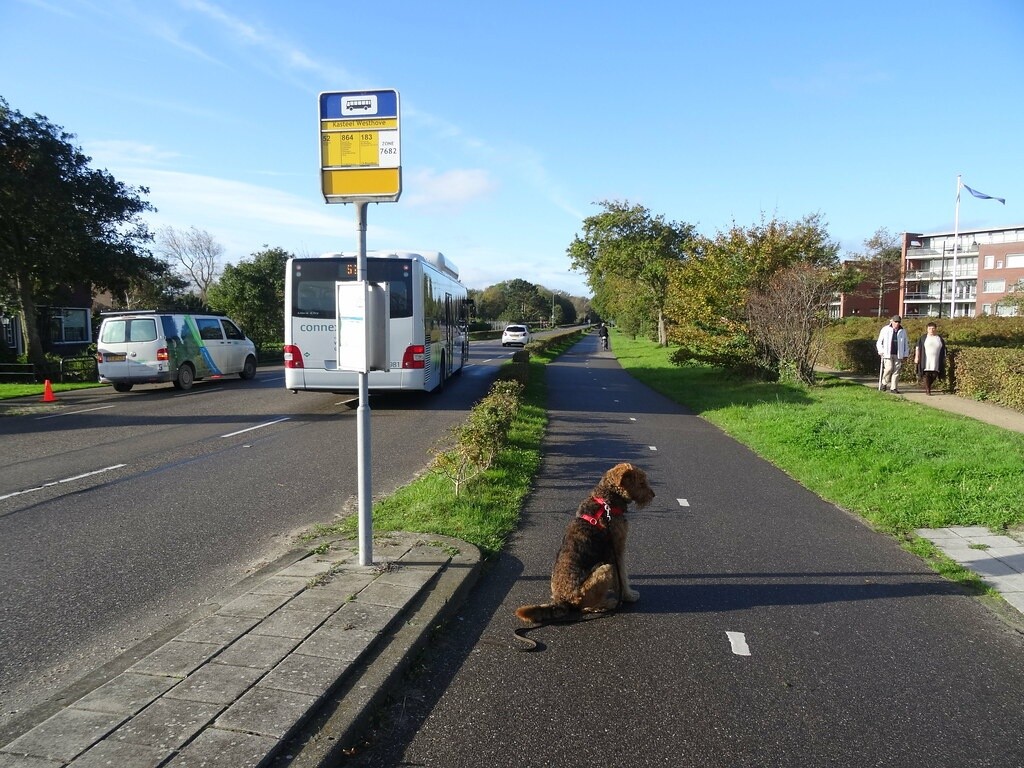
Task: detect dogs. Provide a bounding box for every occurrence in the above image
[516,462,656,624]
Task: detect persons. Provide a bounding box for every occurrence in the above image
[877,315,908,393]
[543,322,549,328]
[598,323,608,349]
[223,323,236,335]
[915,322,946,396]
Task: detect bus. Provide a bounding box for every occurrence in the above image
[284,251,473,394]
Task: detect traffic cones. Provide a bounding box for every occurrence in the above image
[40,380,59,402]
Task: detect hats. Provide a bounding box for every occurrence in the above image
[893,315,902,322]
[601,323,605,327]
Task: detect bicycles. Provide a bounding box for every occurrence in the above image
[598,334,608,352]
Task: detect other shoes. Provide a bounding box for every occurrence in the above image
[891,389,899,393]
[925,387,932,396]
[882,383,887,392]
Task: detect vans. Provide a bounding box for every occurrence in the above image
[97,310,257,394]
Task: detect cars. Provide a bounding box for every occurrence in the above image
[501,325,534,347]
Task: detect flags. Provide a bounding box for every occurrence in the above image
[961,184,1006,204]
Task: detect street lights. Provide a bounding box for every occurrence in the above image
[552,292,561,317]
